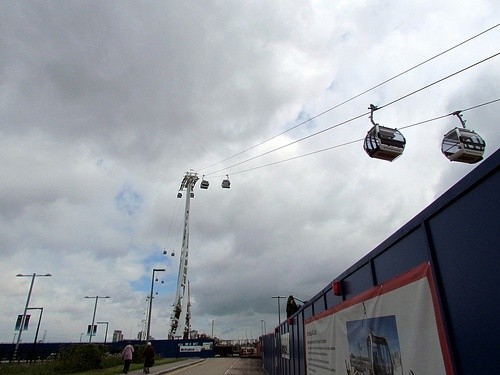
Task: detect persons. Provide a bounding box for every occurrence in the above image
[143,341,153,374]
[121,341,134,374]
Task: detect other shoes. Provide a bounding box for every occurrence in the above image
[143,370,147,374]
[147,370,149,373]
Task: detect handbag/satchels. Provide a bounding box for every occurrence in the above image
[121,355,124,360]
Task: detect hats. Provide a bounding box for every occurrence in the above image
[147,342,151,345]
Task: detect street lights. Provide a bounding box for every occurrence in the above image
[271,295,286,326]
[27,307,44,343]
[97,322,109,343]
[147,268,165,341]
[83,295,110,346]
[12,272,53,360]
